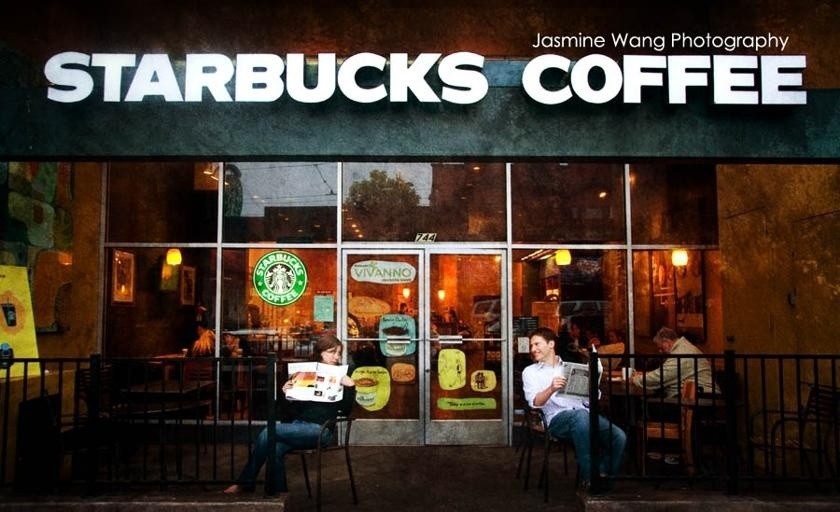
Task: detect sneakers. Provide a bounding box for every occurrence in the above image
[222,483,256,495]
[645,452,682,466]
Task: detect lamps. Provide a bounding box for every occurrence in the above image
[57,251,73,265]
[166,248,182,265]
[671,250,688,266]
[556,250,572,266]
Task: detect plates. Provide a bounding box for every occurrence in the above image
[608,377,625,383]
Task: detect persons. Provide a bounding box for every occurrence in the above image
[224,335,355,494]
[192,321,215,357]
[553,322,625,371]
[522,327,628,490]
[628,326,722,399]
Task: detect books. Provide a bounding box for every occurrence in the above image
[286,362,349,403]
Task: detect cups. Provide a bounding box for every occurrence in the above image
[181,349,189,356]
[382,326,409,357]
[354,377,380,405]
[621,367,637,379]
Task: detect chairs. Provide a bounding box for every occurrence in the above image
[631,377,701,482]
[516,387,583,505]
[284,390,362,512]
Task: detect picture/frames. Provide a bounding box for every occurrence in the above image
[180,264,201,307]
[112,250,137,303]
